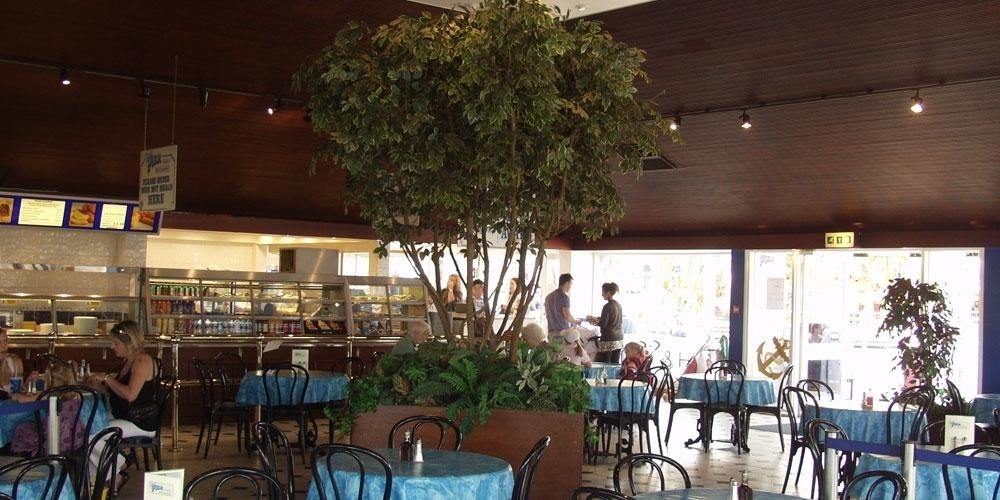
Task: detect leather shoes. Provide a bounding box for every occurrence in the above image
[117,457,132,491]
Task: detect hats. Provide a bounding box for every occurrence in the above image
[560,329,580,342]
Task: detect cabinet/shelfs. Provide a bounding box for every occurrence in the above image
[0,263,434,339]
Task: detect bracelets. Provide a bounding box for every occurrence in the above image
[103,373,111,381]
[447,288,454,292]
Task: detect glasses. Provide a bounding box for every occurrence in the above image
[112,324,127,335]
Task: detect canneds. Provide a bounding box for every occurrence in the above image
[149,284,206,297]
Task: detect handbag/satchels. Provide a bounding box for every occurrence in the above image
[10,398,86,457]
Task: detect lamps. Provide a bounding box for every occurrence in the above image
[670,88,923,130]
[59,72,285,115]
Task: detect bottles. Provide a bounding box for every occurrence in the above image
[192,318,253,334]
[414,439,425,462]
[399,429,413,462]
[866,388,874,407]
[725,476,739,500]
[738,469,754,500]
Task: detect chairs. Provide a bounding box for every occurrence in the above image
[842,470,908,500]
[804,417,852,499]
[573,487,631,498]
[0,353,182,500]
[326,356,364,443]
[613,454,691,495]
[742,364,796,453]
[263,363,317,461]
[795,380,835,487]
[885,386,935,444]
[514,437,550,500]
[594,371,657,469]
[661,360,705,444]
[577,354,663,391]
[310,443,393,498]
[192,355,250,453]
[710,359,747,375]
[941,444,1000,500]
[923,421,988,448]
[184,469,292,499]
[701,368,740,454]
[391,415,462,451]
[782,387,826,498]
[945,379,990,440]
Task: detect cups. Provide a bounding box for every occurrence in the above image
[9,376,22,393]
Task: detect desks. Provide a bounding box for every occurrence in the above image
[238,368,352,408]
[677,373,776,452]
[800,399,930,446]
[585,377,657,468]
[634,487,807,500]
[852,452,1000,499]
[308,450,512,499]
[970,393,1000,424]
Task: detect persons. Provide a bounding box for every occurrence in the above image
[88,319,163,497]
[544,273,582,340]
[617,341,649,378]
[437,274,463,332]
[0,327,23,403]
[500,277,525,315]
[586,281,624,363]
[472,278,487,337]
[7,360,88,457]
[559,327,592,368]
[391,318,432,355]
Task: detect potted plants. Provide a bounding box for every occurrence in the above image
[292,1,687,500]
